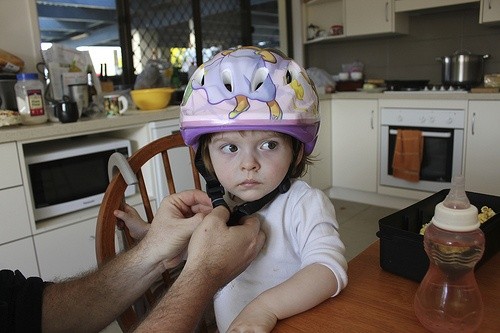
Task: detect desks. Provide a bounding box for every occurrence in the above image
[272,239,500,333]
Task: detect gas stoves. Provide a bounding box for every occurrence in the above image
[383,85,468,94]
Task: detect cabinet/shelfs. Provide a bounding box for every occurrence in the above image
[301,0,500,45]
[1,96,500,279]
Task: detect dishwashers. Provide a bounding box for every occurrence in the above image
[150,118,206,217]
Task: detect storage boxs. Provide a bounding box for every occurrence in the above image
[375,187,500,282]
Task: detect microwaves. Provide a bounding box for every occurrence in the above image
[25,135,138,223]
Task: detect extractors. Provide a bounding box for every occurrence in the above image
[394,0,480,17]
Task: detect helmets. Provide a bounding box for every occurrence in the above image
[179,47,320,156]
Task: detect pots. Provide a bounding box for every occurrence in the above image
[385,78,430,91]
[435,49,490,91]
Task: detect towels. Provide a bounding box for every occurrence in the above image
[392,129,424,183]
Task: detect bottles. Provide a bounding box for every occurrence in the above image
[14,72,48,126]
[307,23,315,40]
[86,71,97,108]
[413,175,485,333]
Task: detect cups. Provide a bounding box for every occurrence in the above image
[68,82,89,115]
[54,101,79,124]
[103,94,128,118]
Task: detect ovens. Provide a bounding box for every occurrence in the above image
[379,107,465,192]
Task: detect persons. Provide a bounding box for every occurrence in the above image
[0,190,265,333]
[112,45,349,333]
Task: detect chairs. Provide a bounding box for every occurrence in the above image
[93,132,205,333]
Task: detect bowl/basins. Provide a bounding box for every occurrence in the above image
[339,72,349,81]
[129,87,175,111]
[351,72,362,81]
